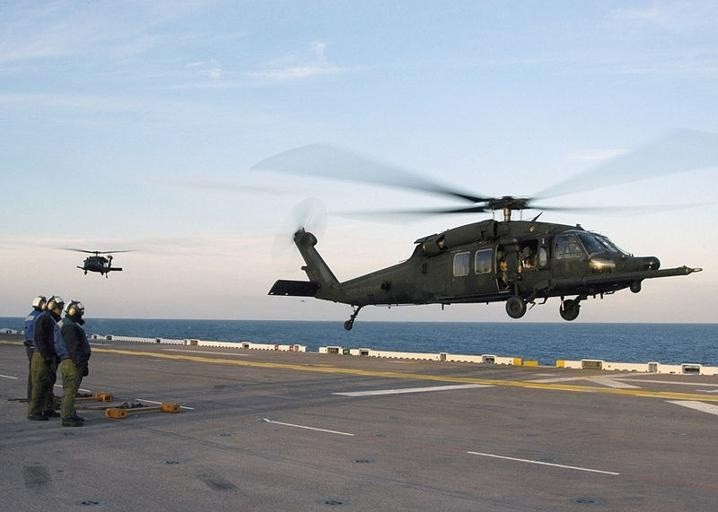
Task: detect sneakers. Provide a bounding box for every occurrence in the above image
[62,415,84,427]
[27,394,61,420]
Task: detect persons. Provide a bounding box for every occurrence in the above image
[497,250,510,291]
[53,299,91,425]
[24,296,48,402]
[27,296,65,421]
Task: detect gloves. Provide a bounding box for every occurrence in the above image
[78,366,88,376]
[45,356,61,364]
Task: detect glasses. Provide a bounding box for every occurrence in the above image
[53,304,63,309]
[76,308,84,315]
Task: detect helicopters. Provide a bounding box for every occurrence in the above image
[65,248,129,274]
[258,150,704,330]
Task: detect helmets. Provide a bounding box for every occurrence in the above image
[45,296,64,310]
[32,296,47,310]
[65,300,84,316]
